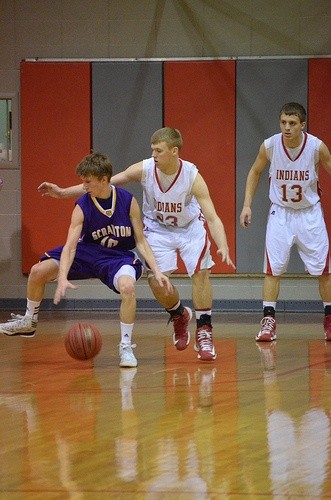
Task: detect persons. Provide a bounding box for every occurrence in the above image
[37,127,236,361]
[0,152,173,366]
[240,102,331,342]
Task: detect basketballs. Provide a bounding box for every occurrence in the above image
[65,323,102,362]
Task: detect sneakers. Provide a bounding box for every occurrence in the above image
[193,324,217,361]
[256,314,278,341]
[0,313,38,338]
[119,367,137,388]
[324,315,331,340]
[117,343,138,367]
[256,341,280,370]
[167,306,192,351]
